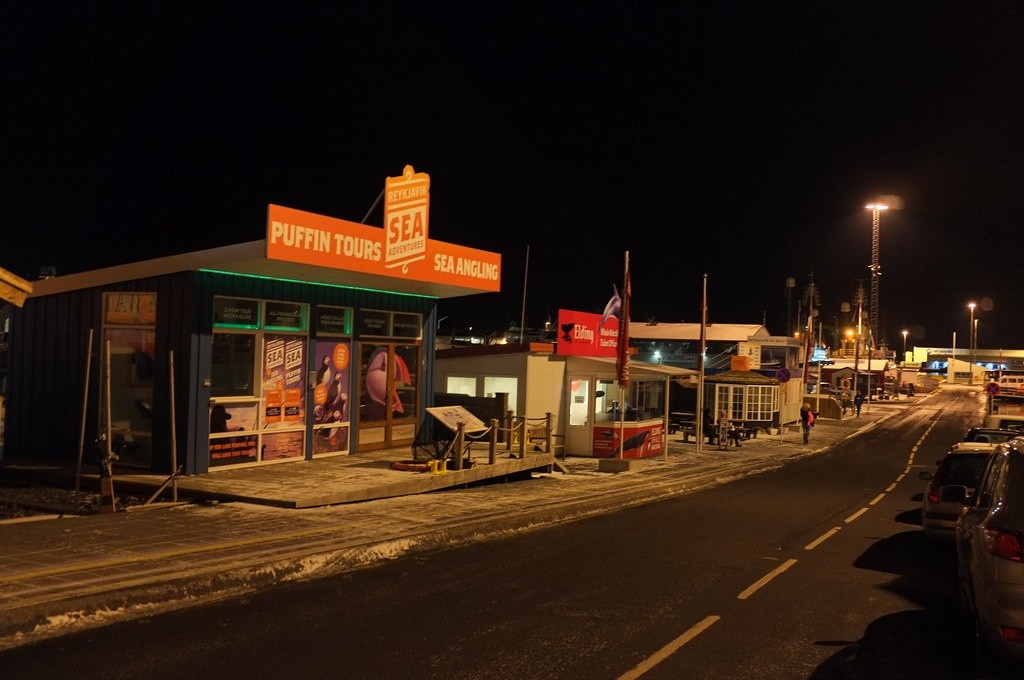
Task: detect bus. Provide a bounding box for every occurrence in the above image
[980,368,1024,390]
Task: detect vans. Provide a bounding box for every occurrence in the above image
[995,376,1024,390]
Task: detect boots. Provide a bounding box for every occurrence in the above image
[736,439,743,447]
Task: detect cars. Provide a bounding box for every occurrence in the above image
[918,442,1002,540]
[981,415,1024,431]
[990,397,1024,418]
[962,426,1024,448]
[993,386,1017,395]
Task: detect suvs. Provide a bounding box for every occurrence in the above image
[957,434,1024,680]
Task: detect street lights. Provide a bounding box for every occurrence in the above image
[844,328,864,401]
[968,302,974,384]
[900,331,909,360]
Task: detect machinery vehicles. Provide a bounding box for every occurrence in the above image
[830,187,897,360]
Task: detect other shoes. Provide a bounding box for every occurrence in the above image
[804,441,809,445]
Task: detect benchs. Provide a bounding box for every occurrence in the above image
[667,411,759,445]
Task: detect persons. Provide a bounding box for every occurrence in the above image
[854,390,864,418]
[716,409,749,447]
[702,408,716,446]
[799,401,815,445]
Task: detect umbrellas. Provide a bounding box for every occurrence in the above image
[615,251,631,460]
